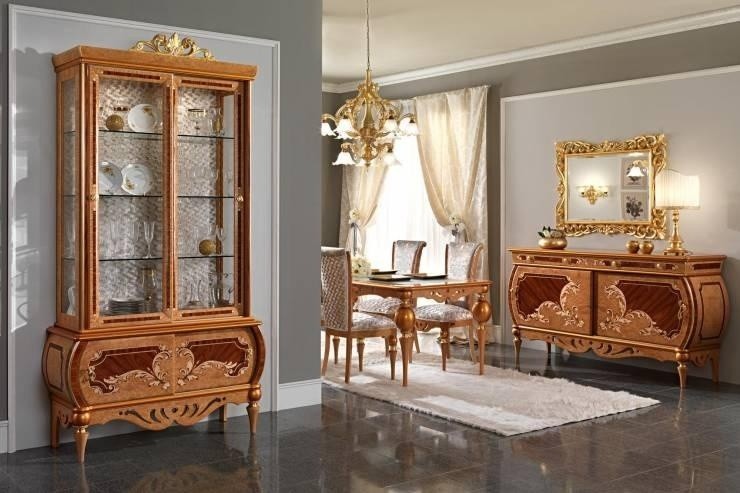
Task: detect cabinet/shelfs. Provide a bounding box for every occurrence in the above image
[506,247,730,389]
[41,31,266,465]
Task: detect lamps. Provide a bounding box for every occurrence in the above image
[321,0,421,170]
[626,163,645,185]
[577,186,609,204]
[653,168,700,257]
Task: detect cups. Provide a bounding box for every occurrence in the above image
[65,284,77,315]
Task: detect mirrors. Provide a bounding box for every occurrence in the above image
[556,132,668,241]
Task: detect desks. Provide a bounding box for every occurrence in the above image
[351,271,493,386]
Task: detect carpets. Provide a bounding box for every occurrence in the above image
[320,331,663,436]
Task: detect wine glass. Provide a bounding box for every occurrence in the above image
[109,219,156,258]
[183,165,220,196]
[188,103,224,136]
[179,224,227,256]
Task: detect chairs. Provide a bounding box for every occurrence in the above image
[385,241,426,364]
[320,244,398,385]
[408,242,493,375]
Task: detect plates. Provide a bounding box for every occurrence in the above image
[119,162,154,194]
[369,269,447,281]
[97,162,123,194]
[107,297,145,315]
[127,103,163,132]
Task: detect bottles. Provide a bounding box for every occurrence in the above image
[139,265,159,313]
[186,279,204,307]
